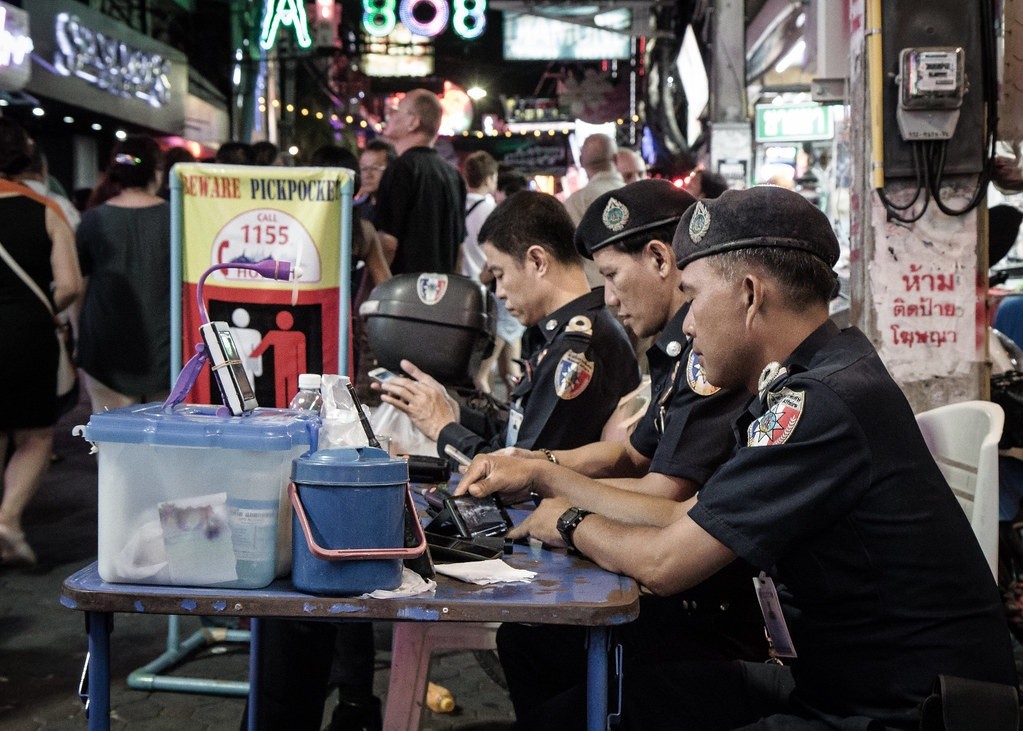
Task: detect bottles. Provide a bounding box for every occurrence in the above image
[286,374,328,421]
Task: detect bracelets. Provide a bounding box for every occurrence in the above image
[539,448,553,462]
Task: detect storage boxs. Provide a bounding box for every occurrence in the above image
[72,402,323,589]
[359,273,483,378]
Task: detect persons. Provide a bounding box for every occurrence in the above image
[370,189,640,473]
[685,170,728,200]
[458,178,766,731]
[0,130,644,562]
[454,187,1023,731]
[367,88,467,276]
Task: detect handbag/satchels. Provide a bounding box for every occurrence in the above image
[991,370,1023,450]
[318,373,425,456]
[56,299,78,395]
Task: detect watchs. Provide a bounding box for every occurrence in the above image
[557,507,596,553]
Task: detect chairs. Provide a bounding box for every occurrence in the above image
[915,400,1005,585]
[992,328,1023,402]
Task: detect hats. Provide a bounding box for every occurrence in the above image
[574,177,699,261]
[671,184,841,265]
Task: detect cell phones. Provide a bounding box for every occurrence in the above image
[367,367,398,385]
[443,493,515,538]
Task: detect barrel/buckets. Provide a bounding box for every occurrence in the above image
[289,444,428,595]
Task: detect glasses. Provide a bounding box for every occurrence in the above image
[359,164,386,172]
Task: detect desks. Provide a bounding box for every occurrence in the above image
[59,474,641,731]
